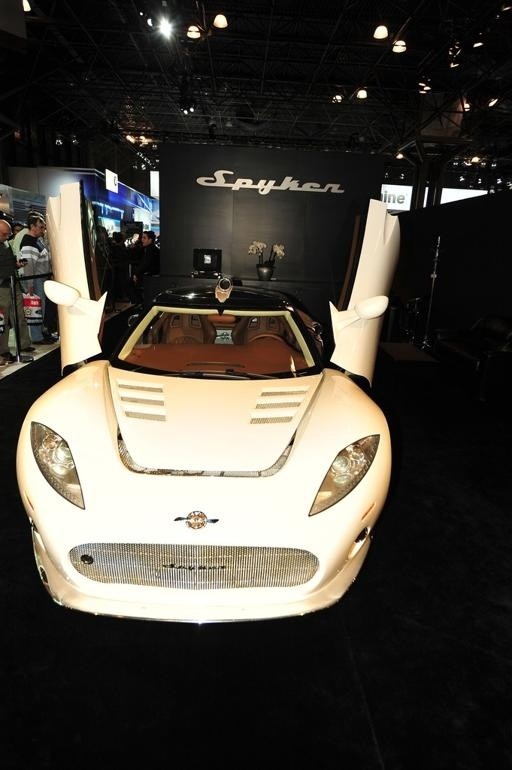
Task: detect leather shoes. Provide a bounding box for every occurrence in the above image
[1,332,57,360]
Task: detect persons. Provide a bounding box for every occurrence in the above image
[1,211,161,368]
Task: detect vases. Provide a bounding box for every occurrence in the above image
[257,268,274,282]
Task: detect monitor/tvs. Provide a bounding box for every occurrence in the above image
[192,248,222,279]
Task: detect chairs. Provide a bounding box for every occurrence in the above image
[148,313,217,344]
[231,316,296,346]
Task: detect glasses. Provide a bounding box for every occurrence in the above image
[38,226,47,229]
[2,233,11,236]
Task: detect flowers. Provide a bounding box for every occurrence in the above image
[250,240,284,267]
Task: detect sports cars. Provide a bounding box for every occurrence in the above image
[15,181,401,622]
